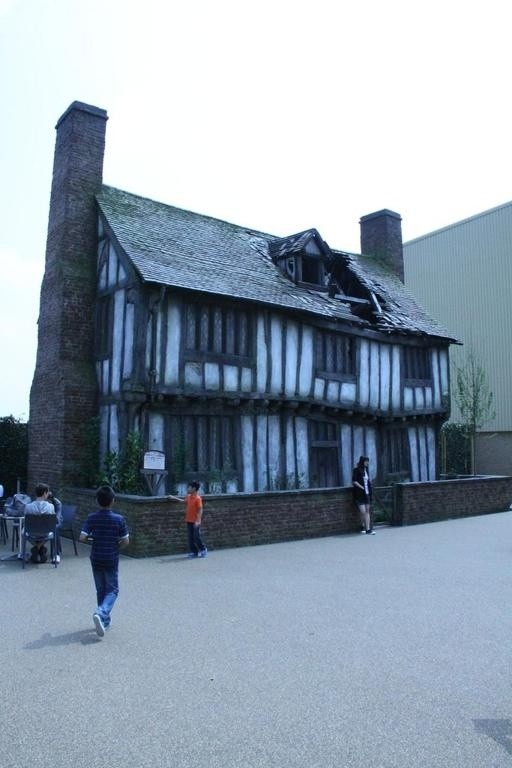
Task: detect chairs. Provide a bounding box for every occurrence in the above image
[22,514,57,569]
[13,515,22,552]
[57,505,79,556]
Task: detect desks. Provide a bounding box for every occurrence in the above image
[0,514,25,561]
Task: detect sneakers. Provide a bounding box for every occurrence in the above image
[361,526,376,535]
[53,555,60,563]
[189,548,207,558]
[31,546,47,562]
[93,614,105,637]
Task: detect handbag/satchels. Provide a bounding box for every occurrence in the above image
[4,494,31,516]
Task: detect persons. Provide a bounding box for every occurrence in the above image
[79,484,130,637]
[21,484,56,563]
[45,485,64,564]
[352,456,376,534]
[168,481,208,558]
